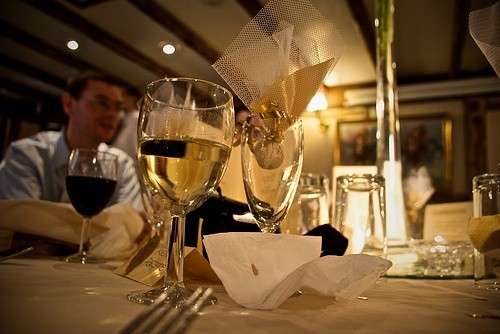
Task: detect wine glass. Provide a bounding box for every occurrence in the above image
[65,148,118,264]
[128,78,235,313]
[141,180,172,242]
[241,114,303,233]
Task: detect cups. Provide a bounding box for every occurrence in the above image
[280,173,328,234]
[411,240,472,275]
[473,174,500,288]
[335,175,386,254]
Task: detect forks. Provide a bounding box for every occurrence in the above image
[120,287,214,334]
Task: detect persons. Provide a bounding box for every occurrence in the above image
[0,72,144,216]
[113,85,146,159]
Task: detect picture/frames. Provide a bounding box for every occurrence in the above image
[332,111,455,203]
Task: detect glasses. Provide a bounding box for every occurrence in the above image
[78,94,127,112]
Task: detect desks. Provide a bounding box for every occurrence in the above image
[424,202,475,245]
